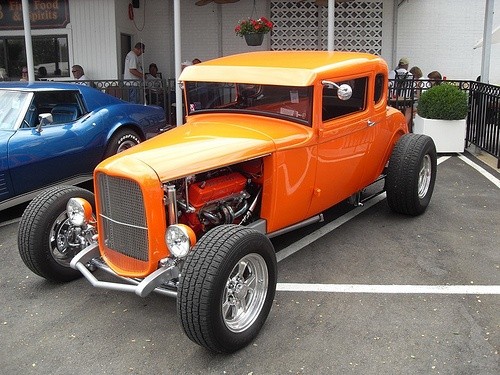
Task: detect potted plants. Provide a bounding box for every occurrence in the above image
[412,82,471,153]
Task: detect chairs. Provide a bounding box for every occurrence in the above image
[19,106,37,129]
[50,106,78,124]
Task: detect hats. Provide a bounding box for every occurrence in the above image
[399,57,409,65]
[137,43,144,53]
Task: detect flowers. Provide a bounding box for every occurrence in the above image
[234,17,273,37]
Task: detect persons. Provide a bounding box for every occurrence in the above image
[123,42,149,103]
[146,63,162,92]
[0,68,8,80]
[387,58,442,98]
[72,65,91,87]
[192,59,201,64]
[21,66,28,81]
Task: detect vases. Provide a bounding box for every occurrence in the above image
[244,32,264,46]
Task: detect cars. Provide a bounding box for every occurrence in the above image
[16,50,437,354]
[0,81,167,212]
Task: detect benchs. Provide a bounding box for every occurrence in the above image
[323,96,356,120]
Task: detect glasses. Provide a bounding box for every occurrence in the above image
[72,70,77,72]
